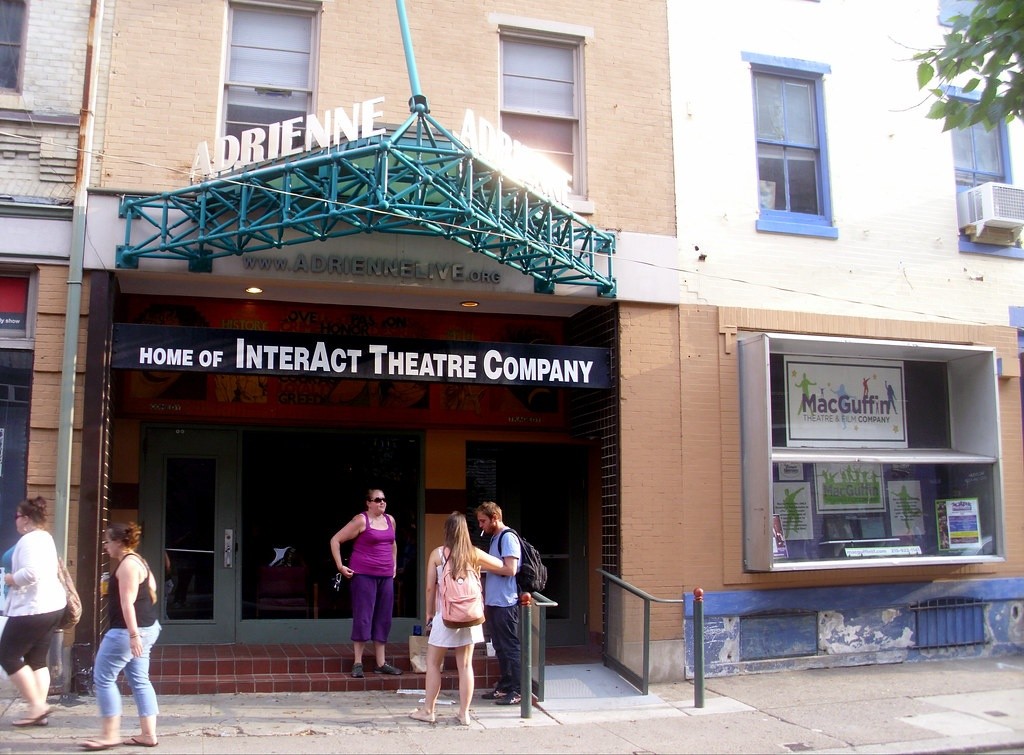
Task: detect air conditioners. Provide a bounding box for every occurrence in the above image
[957,181,1024,230]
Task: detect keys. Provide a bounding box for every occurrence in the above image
[332,572,342,591]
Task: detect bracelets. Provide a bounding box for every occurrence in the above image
[130,633,139,638]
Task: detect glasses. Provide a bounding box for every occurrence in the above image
[101,539,112,546]
[15,514,25,519]
[369,497,387,503]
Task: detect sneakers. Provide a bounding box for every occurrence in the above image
[482,689,507,699]
[351,662,364,678]
[495,691,522,705]
[374,662,403,675]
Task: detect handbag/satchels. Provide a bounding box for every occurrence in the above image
[408,629,446,674]
[57,556,83,630]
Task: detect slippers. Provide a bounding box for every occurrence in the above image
[12,706,54,727]
[82,739,122,750]
[123,738,158,747]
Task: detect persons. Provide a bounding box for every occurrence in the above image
[330,488,403,678]
[477,501,522,705]
[79,523,162,749]
[408,512,504,726]
[0,496,68,727]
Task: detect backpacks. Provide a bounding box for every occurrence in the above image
[438,546,486,629]
[498,529,548,593]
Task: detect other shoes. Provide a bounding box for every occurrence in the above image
[409,710,435,722]
[455,711,470,726]
